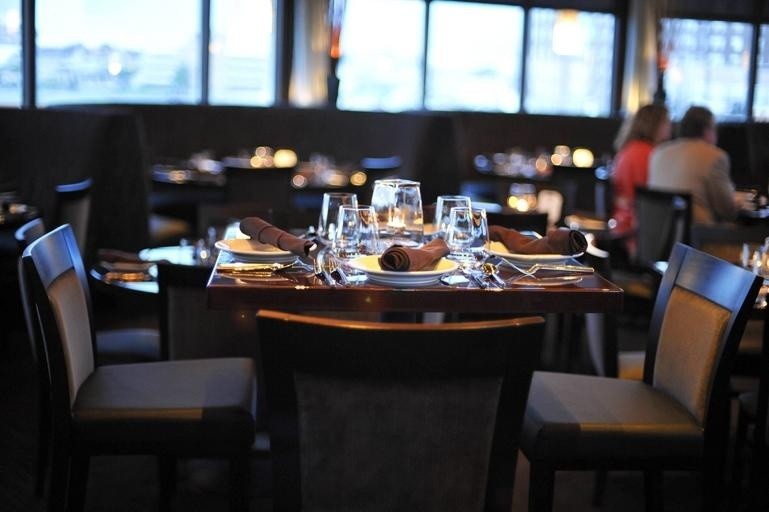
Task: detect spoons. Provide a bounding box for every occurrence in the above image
[463,261,506,288]
[313,254,353,289]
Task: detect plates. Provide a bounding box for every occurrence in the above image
[345,252,461,287]
[214,236,317,258]
[482,241,588,266]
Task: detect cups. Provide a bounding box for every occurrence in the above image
[741,242,769,310]
[436,192,490,261]
[371,177,424,254]
[317,190,380,260]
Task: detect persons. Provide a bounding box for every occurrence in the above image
[610,105,672,256]
[647,106,747,267]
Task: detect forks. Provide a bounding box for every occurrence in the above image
[216,259,316,279]
[496,256,598,277]
